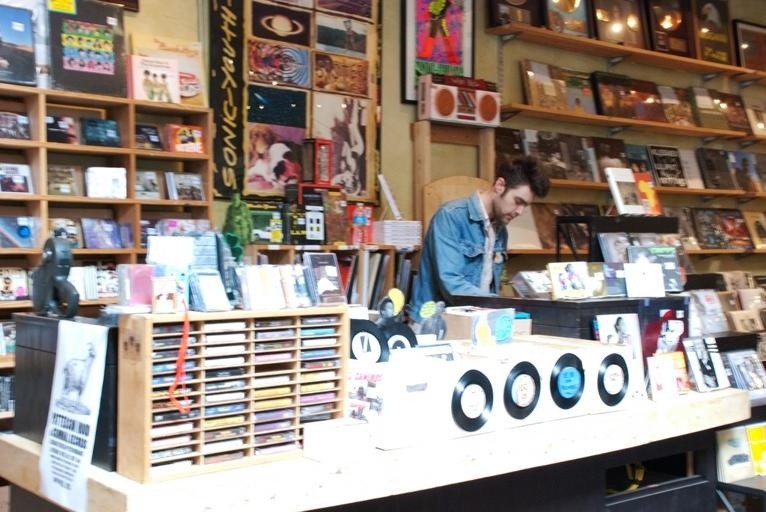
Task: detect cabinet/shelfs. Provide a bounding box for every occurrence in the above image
[0,82,214,423]
[483,20,766,258]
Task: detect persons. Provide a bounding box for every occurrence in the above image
[371,295,398,326]
[418,299,449,340]
[410,154,551,320]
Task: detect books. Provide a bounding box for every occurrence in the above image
[506,167,766,399]
[237,247,347,313]
[1,1,206,108]
[490,0,766,73]
[519,58,766,137]
[715,422,766,482]
[495,126,766,193]
[1,110,235,313]
